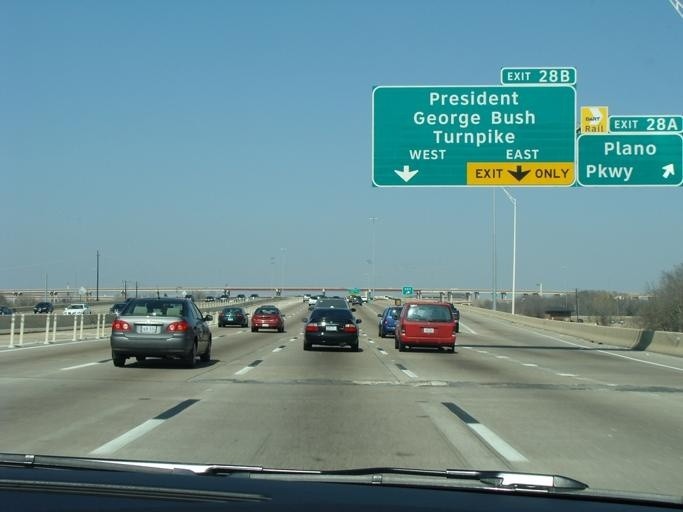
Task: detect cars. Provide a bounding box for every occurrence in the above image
[110,298,213,368]
[250,306,285,332]
[0,306,11,315]
[206,294,258,302]
[109,303,126,313]
[349,295,367,305]
[314,297,356,312]
[33,302,53,313]
[218,307,250,327]
[303,293,327,306]
[185,294,194,302]
[63,303,91,315]
[303,308,361,351]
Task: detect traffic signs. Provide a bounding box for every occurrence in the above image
[578,116,683,187]
[372,66,577,187]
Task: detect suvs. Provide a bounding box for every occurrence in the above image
[378,301,460,352]
